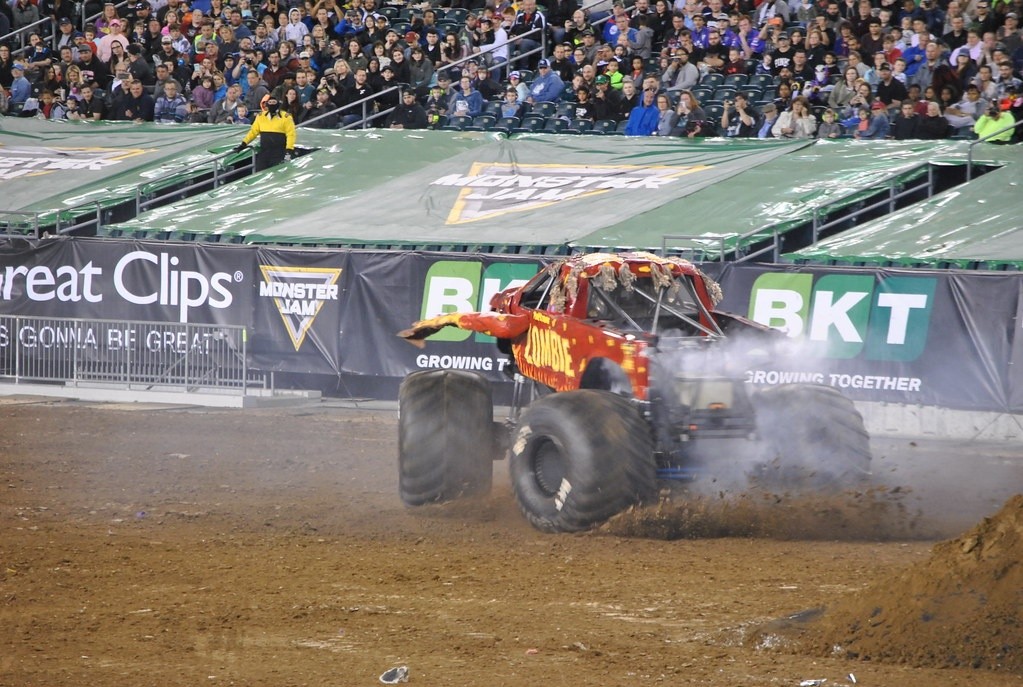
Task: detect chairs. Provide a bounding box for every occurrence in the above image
[378,8,480,45]
[243,19,258,35]
[440,22,845,136]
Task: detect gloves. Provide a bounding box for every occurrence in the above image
[234,142,246,154]
[284,149,293,159]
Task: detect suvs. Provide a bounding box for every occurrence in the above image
[398,253,873,534]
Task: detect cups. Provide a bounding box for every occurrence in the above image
[60,89,65,101]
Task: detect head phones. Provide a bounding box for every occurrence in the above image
[570,15,589,21]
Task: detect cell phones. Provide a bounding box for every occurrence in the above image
[680,102,685,108]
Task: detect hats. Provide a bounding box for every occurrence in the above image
[958,47,970,57]
[614,1,624,7]
[1005,12,1018,19]
[878,63,891,70]
[110,1,147,53]
[161,36,171,45]
[67,95,76,100]
[777,31,788,40]
[299,52,338,94]
[11,64,24,71]
[464,13,491,23]
[692,13,728,21]
[381,65,449,96]
[509,29,607,85]
[762,103,775,112]
[386,28,417,42]
[59,17,91,52]
[205,22,266,45]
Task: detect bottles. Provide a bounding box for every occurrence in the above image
[177,54,184,66]
[430,89,446,97]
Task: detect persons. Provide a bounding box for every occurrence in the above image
[0,0,1023,143]
[233,96,297,172]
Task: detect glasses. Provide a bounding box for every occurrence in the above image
[165,87,177,91]
[976,6,988,9]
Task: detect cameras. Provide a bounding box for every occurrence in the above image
[347,10,357,17]
[444,43,453,55]
[987,99,999,116]
[244,57,253,64]
[727,99,737,105]
